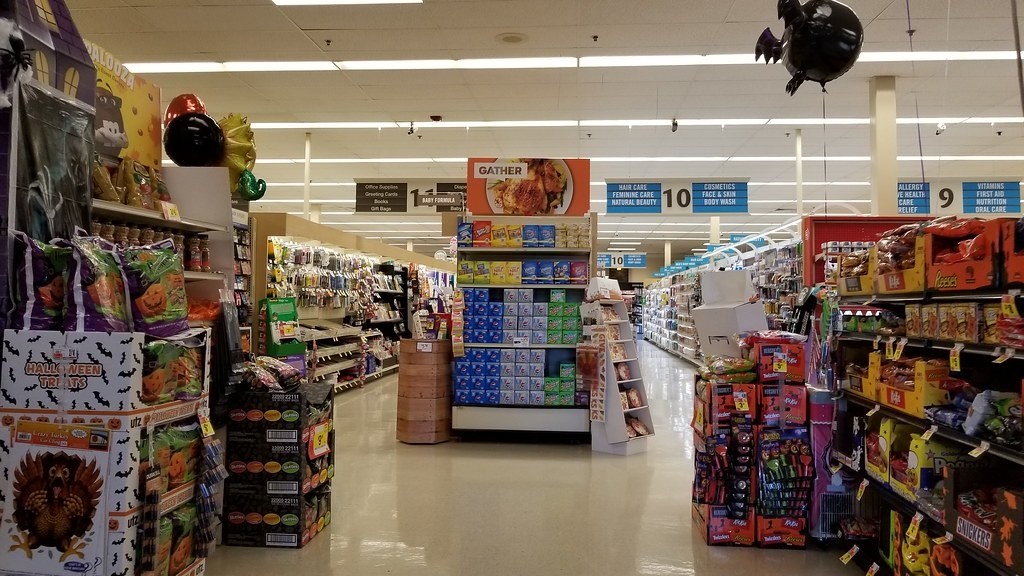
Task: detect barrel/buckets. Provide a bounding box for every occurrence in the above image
[395,339,451,444]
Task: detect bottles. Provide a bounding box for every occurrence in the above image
[90,216,212,272]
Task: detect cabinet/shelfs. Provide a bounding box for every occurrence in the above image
[579,299,657,456]
[638,201,861,367]
[92,199,229,326]
[248,210,457,395]
[452,211,598,440]
[831,294,1024,576]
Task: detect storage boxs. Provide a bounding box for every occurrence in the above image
[0,0,1024,576]
